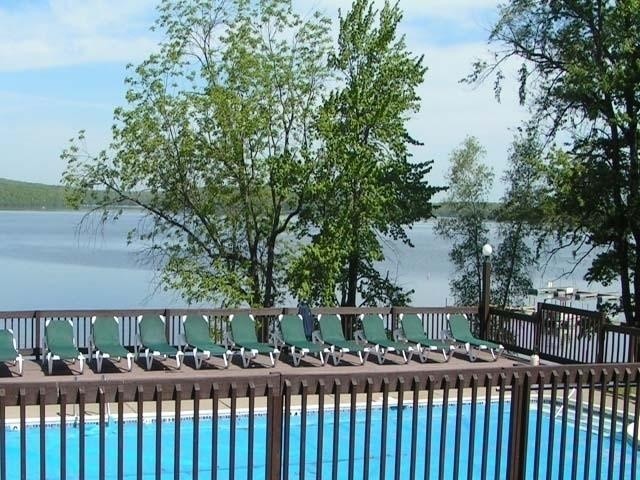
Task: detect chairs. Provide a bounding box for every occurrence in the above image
[274,314,331,367]
[224,313,281,368]
[440,313,505,363]
[392,312,457,363]
[89,316,135,373]
[313,313,371,366]
[134,313,184,371]
[353,314,415,364]
[41,319,86,374]
[0,328,24,376]
[180,314,232,370]
